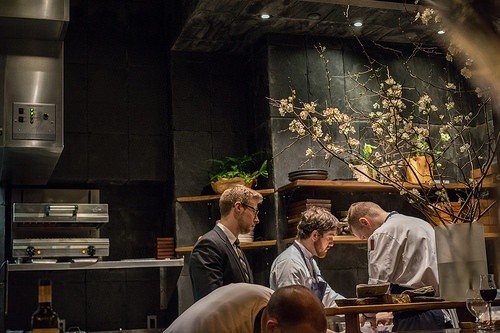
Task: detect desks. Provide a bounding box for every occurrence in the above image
[323,298,500,333]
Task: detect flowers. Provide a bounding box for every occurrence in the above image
[265,5,500,229]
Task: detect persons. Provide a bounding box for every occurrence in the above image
[188,184,262,300]
[347,202,444,333]
[160,282,346,333]
[269,206,367,333]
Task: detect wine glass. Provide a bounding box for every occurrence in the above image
[464,273,498,329]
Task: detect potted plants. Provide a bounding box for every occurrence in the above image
[207,156,268,195]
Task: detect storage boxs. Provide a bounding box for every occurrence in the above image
[403,157,434,184]
[427,202,462,229]
[469,199,500,225]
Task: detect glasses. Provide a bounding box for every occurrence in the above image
[234,203,259,219]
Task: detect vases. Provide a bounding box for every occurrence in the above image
[434,224,489,300]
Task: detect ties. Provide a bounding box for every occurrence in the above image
[234,239,250,283]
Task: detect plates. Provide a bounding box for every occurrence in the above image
[216,219,255,243]
[288,169,328,182]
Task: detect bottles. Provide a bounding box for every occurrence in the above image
[30,277,60,333]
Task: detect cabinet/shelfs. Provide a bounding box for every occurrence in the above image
[278,180,500,244]
[175,188,277,254]
